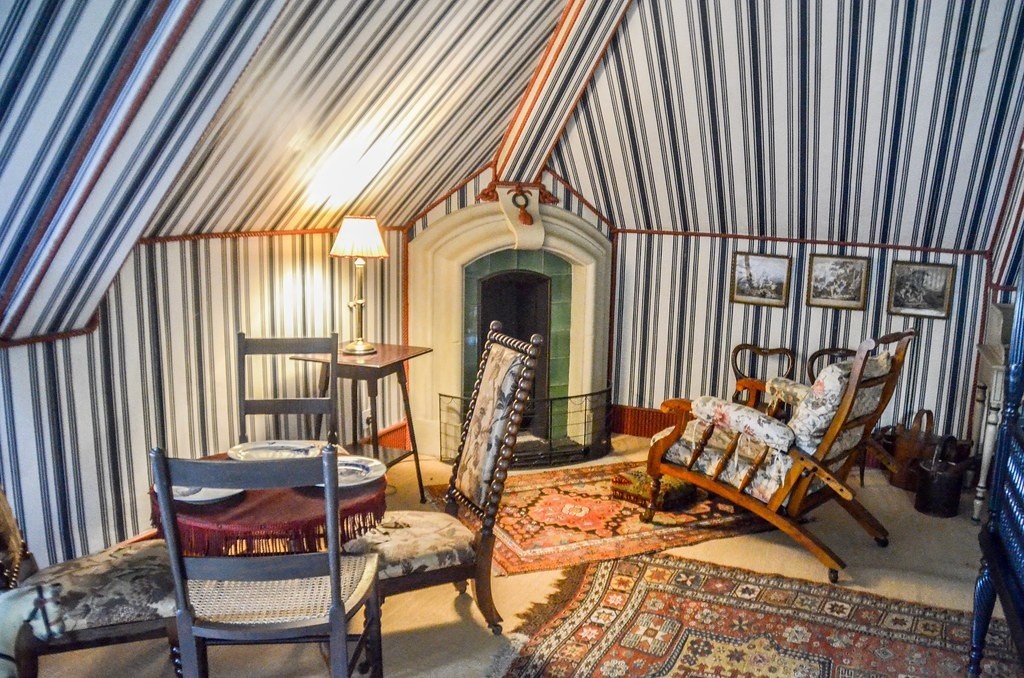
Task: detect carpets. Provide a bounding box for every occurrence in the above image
[474,553,1024,678]
[424,460,805,577]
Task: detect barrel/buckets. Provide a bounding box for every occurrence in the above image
[915,434,963,518]
[889,408,941,493]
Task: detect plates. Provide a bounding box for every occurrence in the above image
[152,482,244,504]
[316,455,386,488]
[227,440,320,461]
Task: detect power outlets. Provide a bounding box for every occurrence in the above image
[361,408,372,431]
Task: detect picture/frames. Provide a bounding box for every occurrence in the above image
[805,253,871,309]
[727,251,791,307]
[888,260,957,319]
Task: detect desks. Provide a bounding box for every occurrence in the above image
[148,450,387,558]
[290,345,434,504]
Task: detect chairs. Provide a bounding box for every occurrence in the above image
[808,345,867,488]
[238,331,339,445]
[139,452,383,677]
[0,494,182,678]
[731,343,794,421]
[646,331,920,583]
[330,320,545,635]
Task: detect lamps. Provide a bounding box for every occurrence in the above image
[323,216,390,357]
